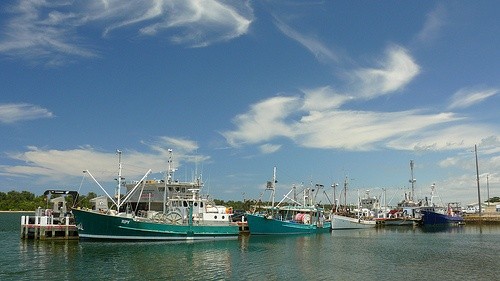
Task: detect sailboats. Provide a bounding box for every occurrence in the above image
[422,183,464,225]
[242,164,333,236]
[330,174,378,230]
[372,185,417,226]
[70,146,240,242]
